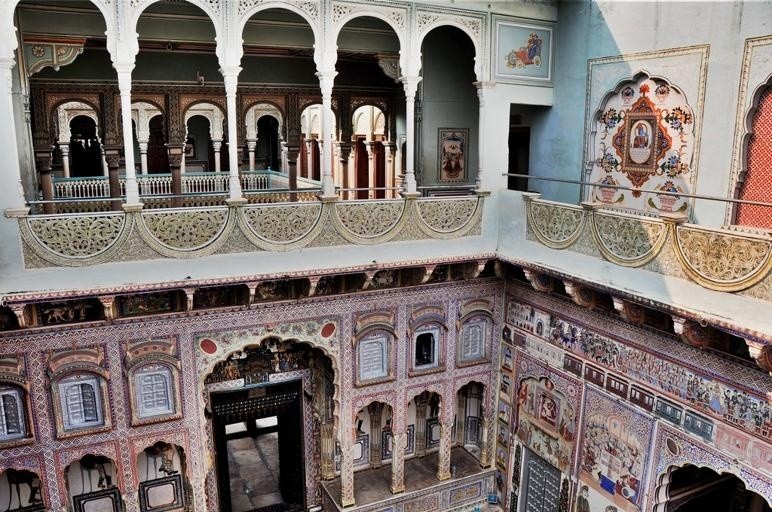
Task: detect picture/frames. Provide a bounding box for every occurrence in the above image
[488,11,558,89]
[435,126,470,186]
[621,110,660,176]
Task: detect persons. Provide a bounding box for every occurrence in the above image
[549,320,772,437]
[518,384,572,471]
[497,450,505,468]
[499,404,509,423]
[498,426,508,445]
[577,486,589,511]
[584,421,638,503]
